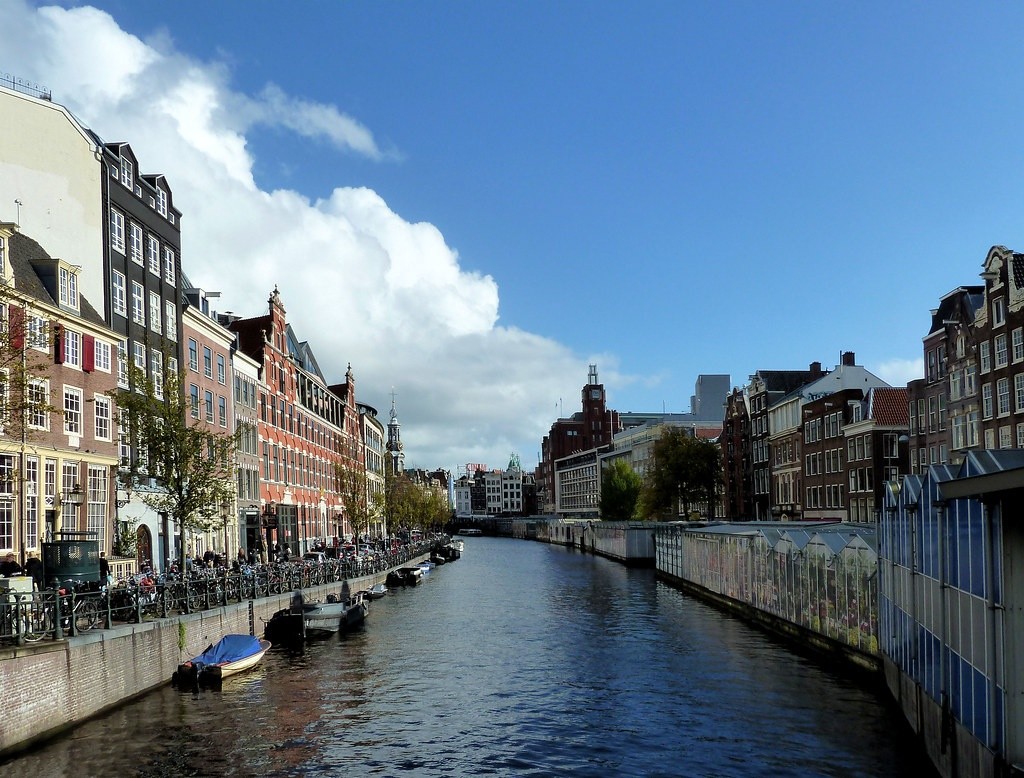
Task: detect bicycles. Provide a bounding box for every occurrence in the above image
[0,552,389,643]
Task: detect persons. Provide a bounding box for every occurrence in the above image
[99,531,383,586]
[24,551,42,592]
[0,552,22,577]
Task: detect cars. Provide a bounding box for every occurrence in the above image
[303,543,375,564]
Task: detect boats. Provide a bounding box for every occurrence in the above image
[178,634,272,679]
[458,528,482,535]
[357,583,388,599]
[392,540,465,587]
[282,599,369,642]
